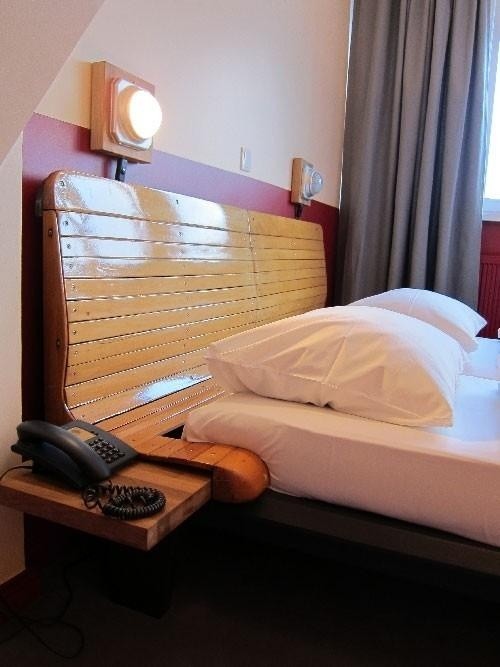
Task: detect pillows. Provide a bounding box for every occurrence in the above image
[203,305,471,427]
[349,288,487,353]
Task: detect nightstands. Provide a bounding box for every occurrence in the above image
[0,463,211,619]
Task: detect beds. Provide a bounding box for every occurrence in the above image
[34,170,500,578]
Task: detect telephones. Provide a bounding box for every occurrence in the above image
[10,420,136,492]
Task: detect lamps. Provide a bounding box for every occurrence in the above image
[291,158,323,206]
[91,61,163,164]
